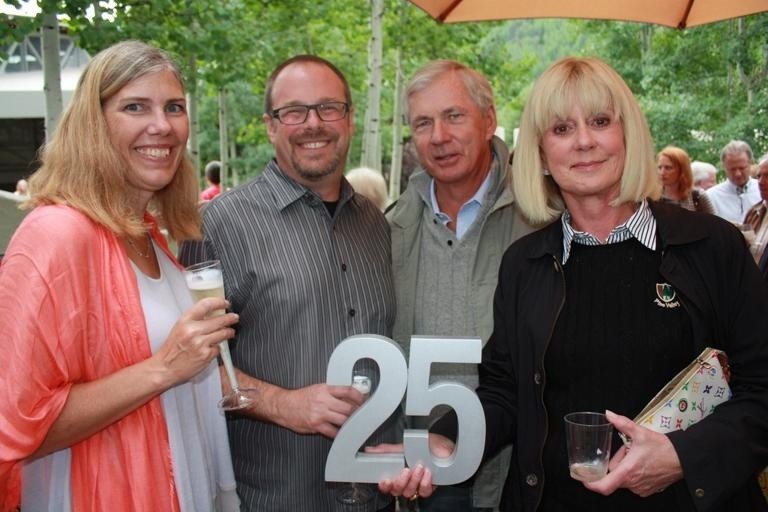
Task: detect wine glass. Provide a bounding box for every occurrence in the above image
[181,259,259,411]
[336,373,375,506]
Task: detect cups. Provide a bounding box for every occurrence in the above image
[563,411,613,483]
[738,222,757,242]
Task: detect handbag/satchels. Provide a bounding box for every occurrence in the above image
[618,346,733,449]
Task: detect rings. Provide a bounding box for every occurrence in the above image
[756,211,760,216]
[408,489,420,501]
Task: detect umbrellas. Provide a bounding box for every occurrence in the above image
[407,0,768,28]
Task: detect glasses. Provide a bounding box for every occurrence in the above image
[268,101,350,126]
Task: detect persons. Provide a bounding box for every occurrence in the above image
[13,178,33,198]
[0,39,248,511]
[363,55,768,512]
[345,166,390,213]
[176,52,399,512]
[689,160,719,189]
[382,59,567,512]
[730,151,768,275]
[198,159,233,204]
[705,138,763,226]
[656,144,717,218]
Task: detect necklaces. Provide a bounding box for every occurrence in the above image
[125,230,154,260]
